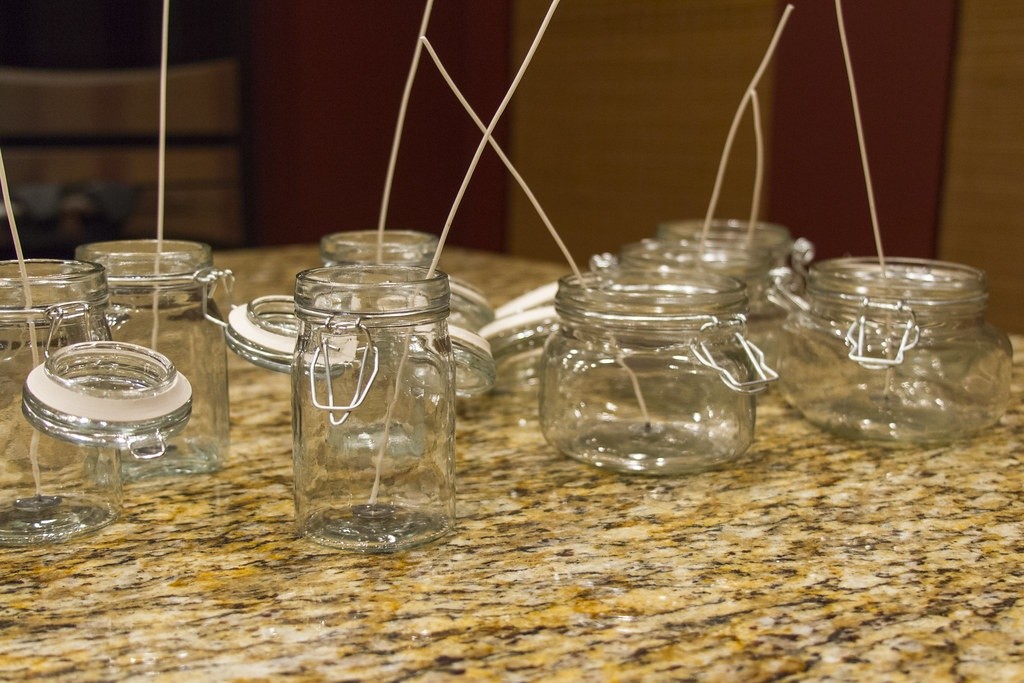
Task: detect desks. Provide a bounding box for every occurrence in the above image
[0,241,1024,683]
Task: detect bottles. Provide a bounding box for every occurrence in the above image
[295,263,495,552]
[2,259,191,547]
[73,238,380,473]
[317,225,497,403]
[484,238,787,408]
[483,241,781,473]
[669,215,1014,451]
